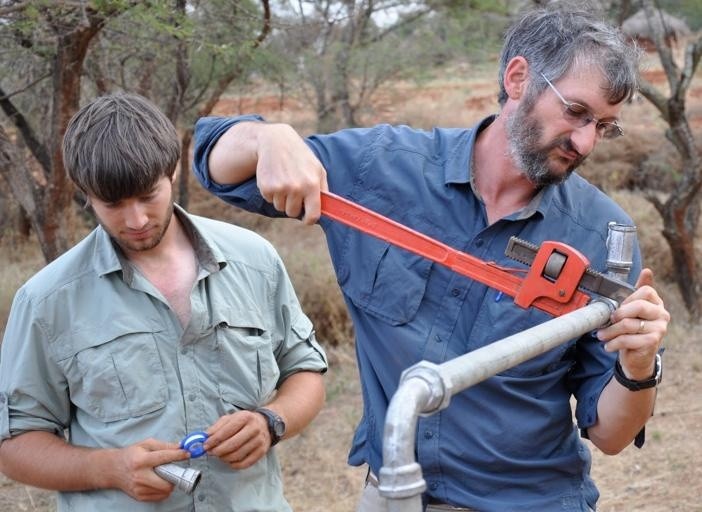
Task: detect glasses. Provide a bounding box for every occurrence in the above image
[529,64,625,141]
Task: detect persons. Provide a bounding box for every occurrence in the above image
[1,91,330,512]
[192,5,672,512]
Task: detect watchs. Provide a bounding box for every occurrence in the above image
[612,350,664,391]
[252,405,286,445]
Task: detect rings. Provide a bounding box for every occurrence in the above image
[639,320,646,335]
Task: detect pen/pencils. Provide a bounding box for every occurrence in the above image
[496,291,502,302]
